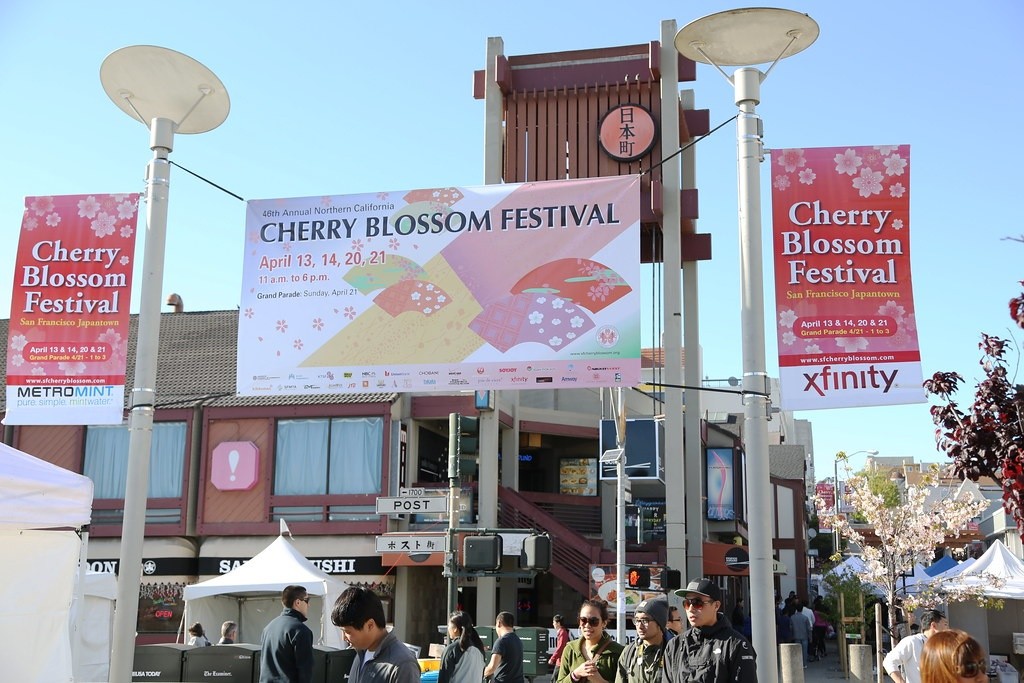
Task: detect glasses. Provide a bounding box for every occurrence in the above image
[668,617,683,624]
[961,658,987,678]
[683,598,714,609]
[578,617,602,627]
[299,597,310,604]
[632,618,655,626]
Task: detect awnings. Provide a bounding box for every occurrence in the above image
[704,542,788,576]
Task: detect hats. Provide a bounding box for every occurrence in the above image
[634,598,669,633]
[674,577,721,601]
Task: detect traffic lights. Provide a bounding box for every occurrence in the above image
[629,567,650,587]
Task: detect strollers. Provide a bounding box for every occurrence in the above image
[807,626,823,662]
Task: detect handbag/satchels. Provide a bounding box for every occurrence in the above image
[825,619,836,639]
[203,635,211,646]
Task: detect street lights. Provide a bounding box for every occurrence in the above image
[100,44,231,683]
[835,450,879,556]
[672,6,820,683]
[892,548,914,594]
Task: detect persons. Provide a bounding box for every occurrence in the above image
[615,599,675,683]
[920,629,988,683]
[882,611,949,683]
[188,622,206,646]
[436,613,485,683]
[661,578,758,683]
[331,587,421,683]
[775,591,831,668]
[483,611,524,683]
[259,586,313,683]
[732,598,751,644]
[218,621,237,644]
[548,615,570,683]
[555,600,624,683]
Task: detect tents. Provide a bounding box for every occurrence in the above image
[820,539,1024,673]
[0,442,117,683]
[183,536,348,649]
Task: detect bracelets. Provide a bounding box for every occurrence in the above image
[571,672,579,681]
